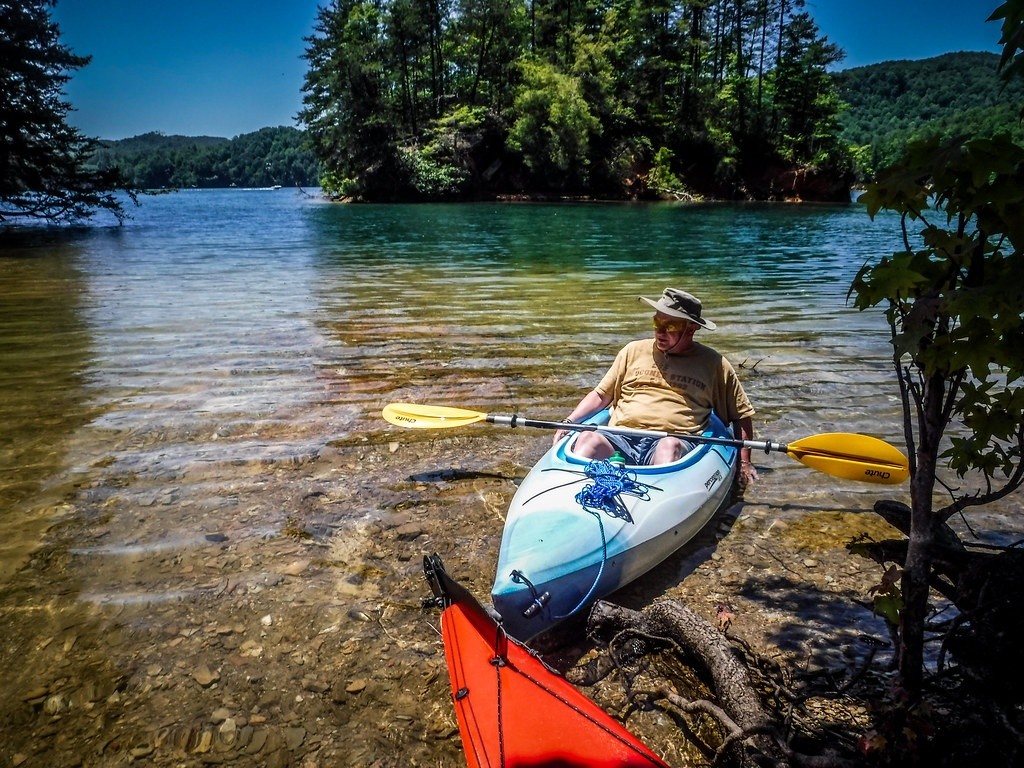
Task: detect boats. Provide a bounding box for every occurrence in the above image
[422,556,671,768]
[491,404,743,647]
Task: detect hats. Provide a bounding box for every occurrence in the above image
[639,288,717,331]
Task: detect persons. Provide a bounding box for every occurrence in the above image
[553,287,757,472]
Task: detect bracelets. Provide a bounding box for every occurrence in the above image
[560,418,572,424]
[739,460,752,465]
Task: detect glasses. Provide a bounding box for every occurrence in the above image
[652,314,691,333]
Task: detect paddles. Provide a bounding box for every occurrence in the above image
[382,402,909,487]
